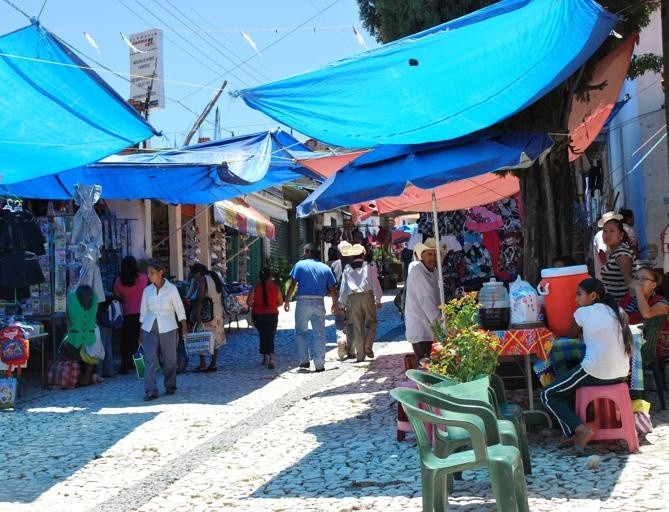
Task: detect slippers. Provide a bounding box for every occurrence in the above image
[262,360,274,369]
[194,366,217,373]
[557,436,594,457]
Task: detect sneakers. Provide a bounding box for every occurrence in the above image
[299,363,309,368]
[316,368,325,372]
[337,343,374,362]
[144,394,155,401]
[166,388,176,395]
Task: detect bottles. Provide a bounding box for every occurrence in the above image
[478,278,511,331]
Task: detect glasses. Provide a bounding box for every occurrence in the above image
[636,277,656,283]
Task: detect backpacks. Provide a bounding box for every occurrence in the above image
[0,327,29,364]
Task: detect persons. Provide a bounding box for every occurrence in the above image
[552,256,577,267]
[617,267,669,400]
[405,238,445,361]
[330,240,356,361]
[284,242,337,372]
[540,278,635,454]
[338,244,383,362]
[593,209,638,311]
[59,255,227,400]
[247,268,283,368]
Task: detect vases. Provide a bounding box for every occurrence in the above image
[430,377,491,440]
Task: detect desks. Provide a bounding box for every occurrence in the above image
[430,324,557,430]
[531,324,649,423]
[16,332,49,399]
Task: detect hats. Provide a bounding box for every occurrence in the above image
[337,241,366,257]
[416,237,446,261]
[598,212,623,227]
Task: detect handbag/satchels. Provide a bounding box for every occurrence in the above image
[223,294,240,317]
[46,359,80,389]
[96,297,125,327]
[189,298,213,323]
[184,333,215,357]
[132,351,161,380]
[79,339,105,365]
[631,399,653,442]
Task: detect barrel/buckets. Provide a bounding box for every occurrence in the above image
[536,265,593,338]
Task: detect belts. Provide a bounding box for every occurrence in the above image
[362,290,374,294]
[298,295,325,299]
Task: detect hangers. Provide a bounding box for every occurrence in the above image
[0,198,32,218]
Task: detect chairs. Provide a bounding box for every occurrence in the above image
[390,386,530,512]
[405,368,532,493]
[630,312,668,414]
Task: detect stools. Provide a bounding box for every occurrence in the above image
[574,382,640,452]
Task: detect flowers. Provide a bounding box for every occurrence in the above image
[419,289,503,385]
